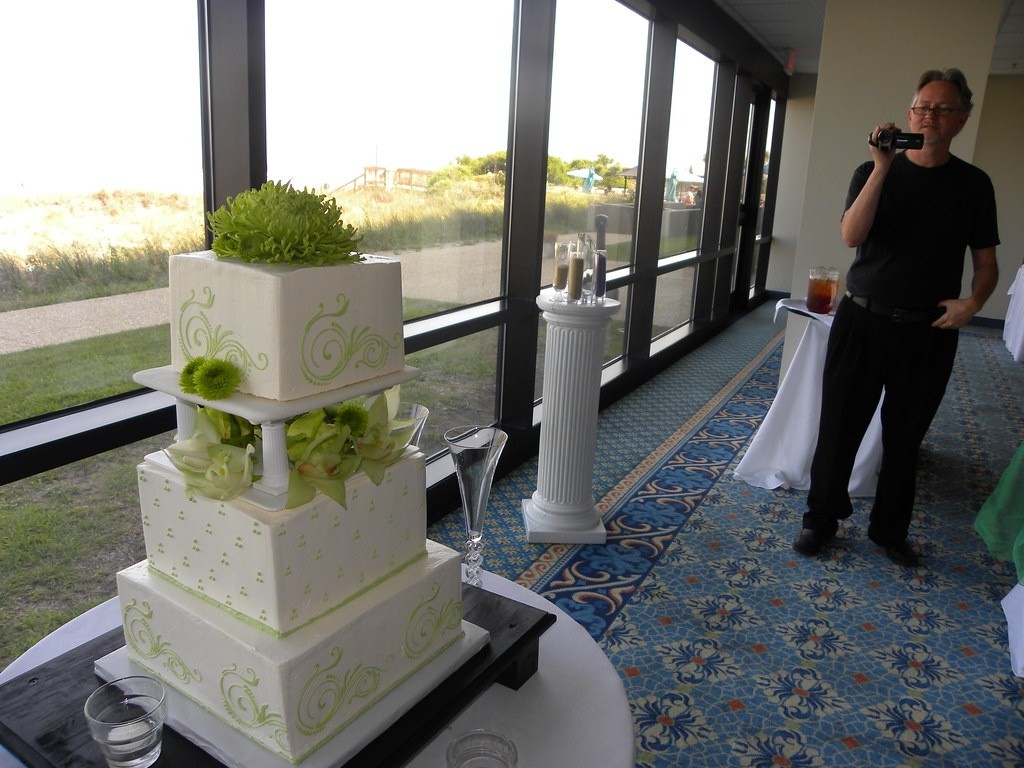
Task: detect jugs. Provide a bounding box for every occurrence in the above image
[806,266,840,314]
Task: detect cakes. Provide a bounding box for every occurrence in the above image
[117,179,466,762]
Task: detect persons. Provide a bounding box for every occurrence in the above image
[793,68,1002,569]
[684,184,703,207]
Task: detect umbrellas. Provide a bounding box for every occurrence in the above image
[566,167,604,180]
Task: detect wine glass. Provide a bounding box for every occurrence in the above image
[444,425,508,586]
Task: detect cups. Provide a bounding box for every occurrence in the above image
[395,402,429,446]
[84,675,165,768]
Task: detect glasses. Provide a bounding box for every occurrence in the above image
[911,107,956,116]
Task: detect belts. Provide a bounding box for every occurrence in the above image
[844,289,946,323]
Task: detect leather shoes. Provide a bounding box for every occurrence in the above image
[867,524,919,567]
[792,528,838,557]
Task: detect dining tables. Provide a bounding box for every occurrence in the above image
[0,563,636,768]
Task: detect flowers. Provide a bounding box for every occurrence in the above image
[157,394,405,512]
[179,358,239,401]
[203,179,364,269]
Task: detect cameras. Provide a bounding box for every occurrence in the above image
[878,127,924,152]
